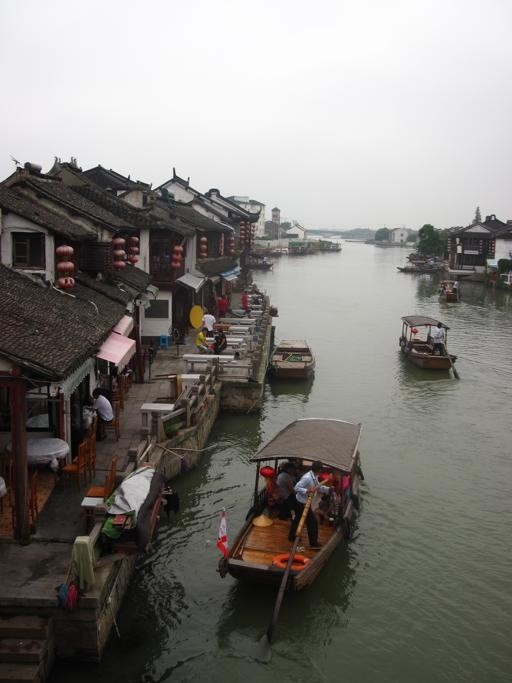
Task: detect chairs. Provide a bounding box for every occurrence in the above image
[100,366,132,440]
[57,415,119,528]
[0,457,39,527]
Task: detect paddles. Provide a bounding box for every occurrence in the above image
[441,337,459,378]
[253,474,319,663]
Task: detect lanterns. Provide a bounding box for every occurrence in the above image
[170,243,182,275]
[478,239,495,255]
[199,236,208,243]
[109,232,143,272]
[447,237,452,252]
[55,244,75,294]
[198,252,208,258]
[260,464,276,479]
[198,244,208,251]
[412,327,418,333]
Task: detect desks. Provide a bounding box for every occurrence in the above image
[6,437,69,472]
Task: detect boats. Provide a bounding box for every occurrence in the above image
[398,314,459,371]
[270,335,316,379]
[246,238,342,269]
[267,378,315,402]
[214,411,369,596]
[397,352,452,382]
[439,278,462,303]
[394,251,444,273]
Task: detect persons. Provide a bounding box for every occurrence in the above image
[196,327,212,354]
[275,462,299,510]
[432,321,445,356]
[211,327,227,354]
[218,294,230,324]
[201,309,217,337]
[238,290,254,318]
[452,278,459,294]
[86,388,114,441]
[287,460,335,548]
[223,217,258,255]
[314,479,342,522]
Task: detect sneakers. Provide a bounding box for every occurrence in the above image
[289,536,321,546]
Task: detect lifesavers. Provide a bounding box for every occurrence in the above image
[273,553,310,571]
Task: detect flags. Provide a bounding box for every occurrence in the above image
[215,506,229,561]
